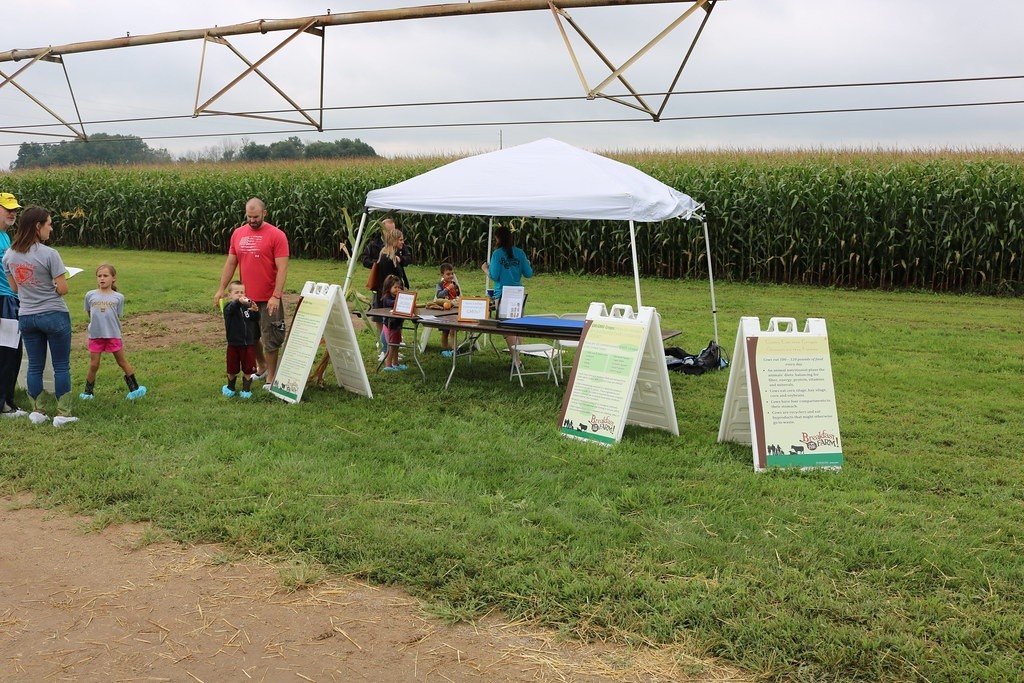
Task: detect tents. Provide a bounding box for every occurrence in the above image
[340,138,719,348]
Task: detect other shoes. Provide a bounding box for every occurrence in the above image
[400,336,407,349]
[376,342,383,349]
[441,349,458,358]
[380,351,406,362]
[392,363,407,371]
[262,384,272,391]
[250,368,268,381]
[384,366,398,372]
[509,361,524,371]
[1,402,27,419]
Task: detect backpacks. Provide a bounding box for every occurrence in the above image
[696,342,729,371]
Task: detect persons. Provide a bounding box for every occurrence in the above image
[0,192,32,417]
[435,262,460,357]
[213,197,292,389]
[3,205,80,428]
[482,226,533,370]
[382,275,408,372]
[222,280,261,397]
[364,219,411,335]
[373,229,406,349]
[77,265,148,400]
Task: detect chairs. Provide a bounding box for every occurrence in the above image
[510,312,662,388]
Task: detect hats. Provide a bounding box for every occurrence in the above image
[0,193,22,209]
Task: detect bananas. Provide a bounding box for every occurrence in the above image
[425,302,443,310]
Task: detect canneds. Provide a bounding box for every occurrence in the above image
[485,289,494,305]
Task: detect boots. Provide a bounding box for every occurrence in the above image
[222,375,237,398]
[53,391,80,428]
[240,376,252,400]
[79,380,95,401]
[124,374,146,399]
[26,391,50,424]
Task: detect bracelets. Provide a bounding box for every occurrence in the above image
[272,291,283,299]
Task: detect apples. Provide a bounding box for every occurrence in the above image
[443,302,451,309]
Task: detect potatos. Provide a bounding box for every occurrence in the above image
[436,299,454,306]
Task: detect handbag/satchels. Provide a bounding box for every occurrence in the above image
[366,262,380,290]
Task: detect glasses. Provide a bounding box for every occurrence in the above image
[0,205,18,213]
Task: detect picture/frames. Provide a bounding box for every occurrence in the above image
[393,290,418,317]
[458,297,490,322]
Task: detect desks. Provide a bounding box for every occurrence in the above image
[417,307,682,390]
[351,306,458,385]
